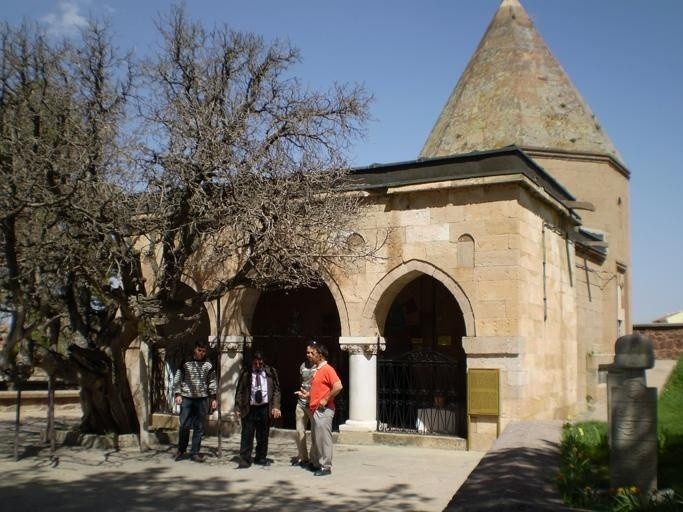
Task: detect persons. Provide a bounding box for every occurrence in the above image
[234,350,281,469]
[292,341,343,475]
[170,341,218,463]
[289,339,322,469]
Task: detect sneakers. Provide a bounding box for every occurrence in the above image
[253,456,271,466]
[238,455,251,468]
[174,452,206,463]
[291,455,332,477]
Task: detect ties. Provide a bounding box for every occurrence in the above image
[254,372,263,404]
